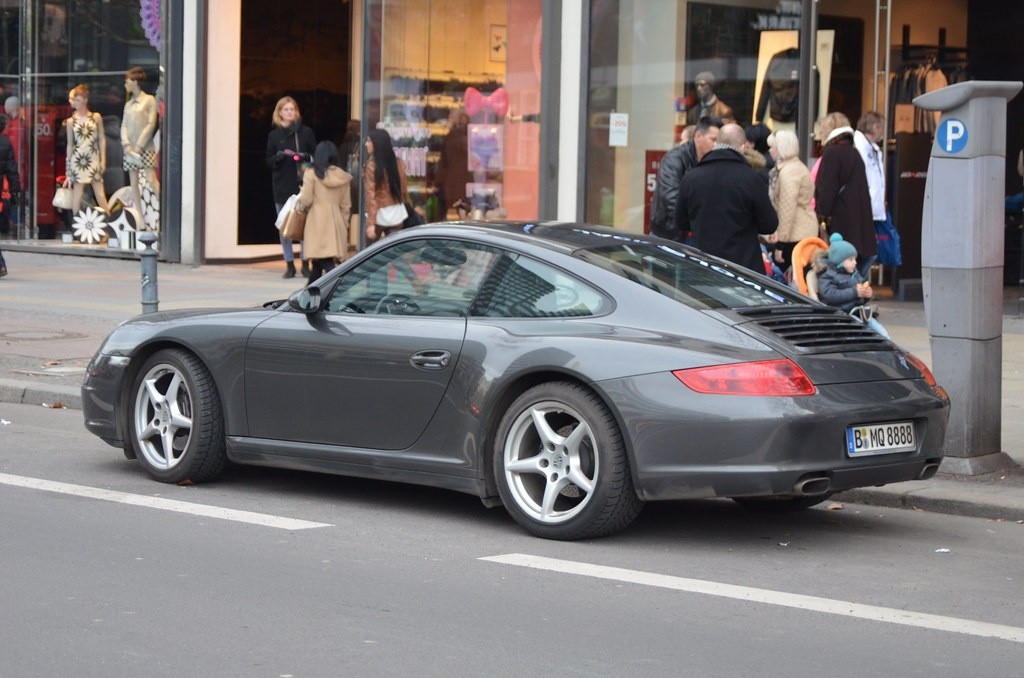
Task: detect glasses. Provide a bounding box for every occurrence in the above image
[695,83,707,88]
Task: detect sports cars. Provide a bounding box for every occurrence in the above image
[80,219,954,543]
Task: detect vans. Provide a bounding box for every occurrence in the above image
[2,94,164,228]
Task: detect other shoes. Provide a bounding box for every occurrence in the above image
[286,268,296,278]
[302,267,311,277]
[0,264,7,276]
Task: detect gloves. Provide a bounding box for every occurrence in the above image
[274,151,284,163]
[10,193,19,204]
[292,153,304,164]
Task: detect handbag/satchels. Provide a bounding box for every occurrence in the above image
[51,177,73,210]
[297,161,311,182]
[274,194,307,239]
[404,201,425,229]
[874,211,902,266]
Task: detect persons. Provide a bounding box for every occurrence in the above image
[685,70,735,129]
[0,134,21,276]
[267,96,320,279]
[650,113,905,339]
[0,97,28,234]
[300,141,353,286]
[437,103,498,213]
[63,66,161,244]
[360,129,426,297]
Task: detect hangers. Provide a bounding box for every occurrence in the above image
[904,54,935,78]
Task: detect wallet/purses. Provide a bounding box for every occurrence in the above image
[126,151,144,168]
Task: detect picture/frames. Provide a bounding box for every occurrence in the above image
[489,24,507,62]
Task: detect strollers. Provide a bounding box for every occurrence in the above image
[759,236,879,321]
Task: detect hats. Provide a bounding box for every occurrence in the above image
[828,232,858,267]
[695,71,716,88]
